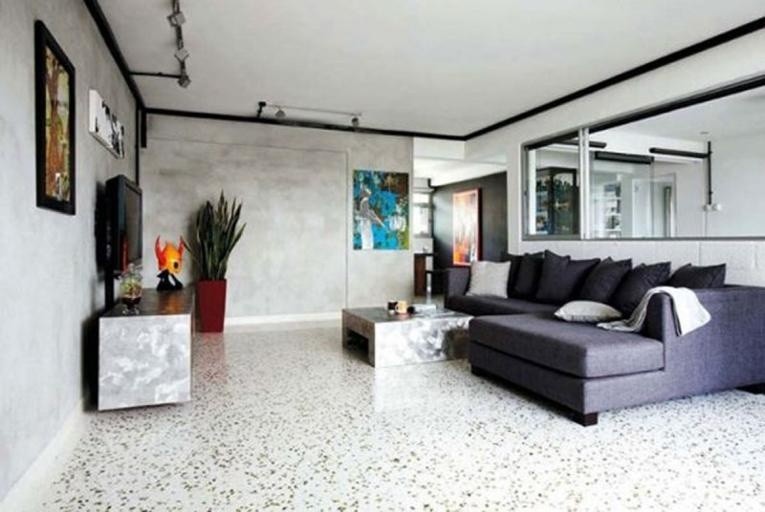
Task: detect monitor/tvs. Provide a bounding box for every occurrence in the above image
[105,175,143,272]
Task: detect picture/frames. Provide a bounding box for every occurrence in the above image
[31,19,75,217]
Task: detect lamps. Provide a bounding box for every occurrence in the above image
[163,11,192,89]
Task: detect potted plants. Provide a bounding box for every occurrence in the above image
[180,187,246,333]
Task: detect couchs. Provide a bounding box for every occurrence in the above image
[443,265,765,426]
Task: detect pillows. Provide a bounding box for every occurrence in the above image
[462,249,728,323]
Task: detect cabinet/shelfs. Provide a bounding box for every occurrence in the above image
[534,166,578,235]
